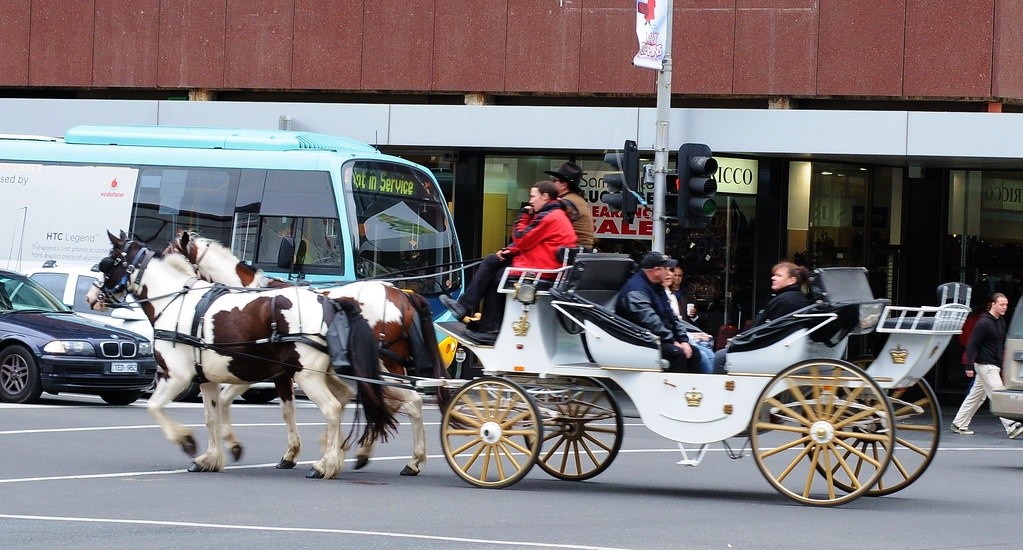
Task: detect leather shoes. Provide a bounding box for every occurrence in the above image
[440,294,469,320]
[465,329,501,345]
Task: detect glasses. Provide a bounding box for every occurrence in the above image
[664,267,675,273]
[550,178,563,184]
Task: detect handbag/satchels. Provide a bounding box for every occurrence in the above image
[676,319,716,348]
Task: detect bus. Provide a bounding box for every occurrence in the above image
[0,123,466,405]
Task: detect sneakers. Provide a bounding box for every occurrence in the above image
[1008,425,1023,439]
[950,424,974,434]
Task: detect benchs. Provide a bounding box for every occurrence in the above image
[887,294,963,331]
[497,244,595,291]
[726,302,855,371]
[582,291,714,368]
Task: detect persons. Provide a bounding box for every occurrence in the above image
[950,292,1023,438]
[267,223,317,266]
[439,181,577,343]
[614,251,716,372]
[542,161,595,251]
[714,261,817,375]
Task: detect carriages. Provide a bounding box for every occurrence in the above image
[86,226,973,508]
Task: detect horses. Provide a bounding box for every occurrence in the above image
[160,227,460,477]
[89,233,400,477]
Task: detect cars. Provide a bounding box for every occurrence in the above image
[0,268,158,406]
[989,296,1023,426]
[8,262,300,403]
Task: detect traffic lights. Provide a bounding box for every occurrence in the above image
[603,139,640,225]
[678,142,719,227]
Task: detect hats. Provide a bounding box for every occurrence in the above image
[639,251,678,269]
[543,162,584,193]
